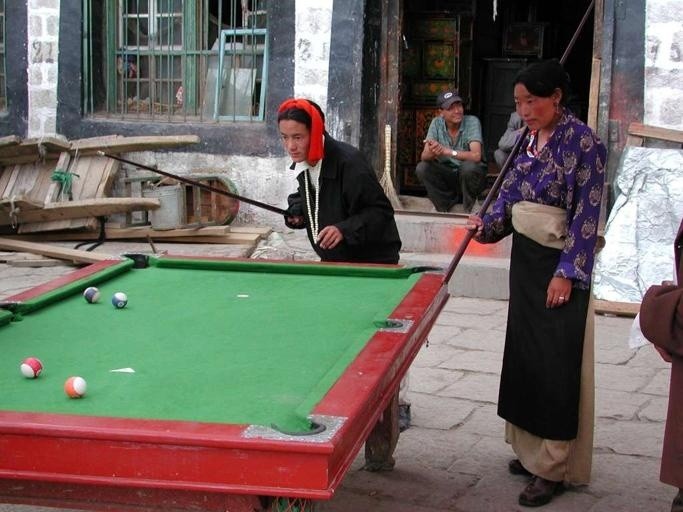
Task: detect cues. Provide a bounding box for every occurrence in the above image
[446,1,594,280]
[96,150,294,218]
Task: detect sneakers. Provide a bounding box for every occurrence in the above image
[397,405,410,432]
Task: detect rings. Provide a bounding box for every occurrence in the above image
[558,294,565,299]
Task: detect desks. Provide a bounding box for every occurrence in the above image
[0,250,451,510]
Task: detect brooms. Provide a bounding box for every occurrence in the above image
[378,123,404,209]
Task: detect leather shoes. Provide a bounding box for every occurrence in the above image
[519,476,563,507]
[509,459,528,473]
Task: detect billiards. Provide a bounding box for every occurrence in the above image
[84,287,100,303]
[64,376,87,398]
[112,292,128,307]
[21,357,41,377]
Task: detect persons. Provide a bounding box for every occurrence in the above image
[276,99,402,267]
[412,91,488,216]
[465,59,606,508]
[492,108,525,174]
[629,215,682,512]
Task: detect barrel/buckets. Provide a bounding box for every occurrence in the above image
[141,177,185,232]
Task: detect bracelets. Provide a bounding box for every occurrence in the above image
[449,148,457,159]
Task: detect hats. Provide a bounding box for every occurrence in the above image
[437,90,461,110]
